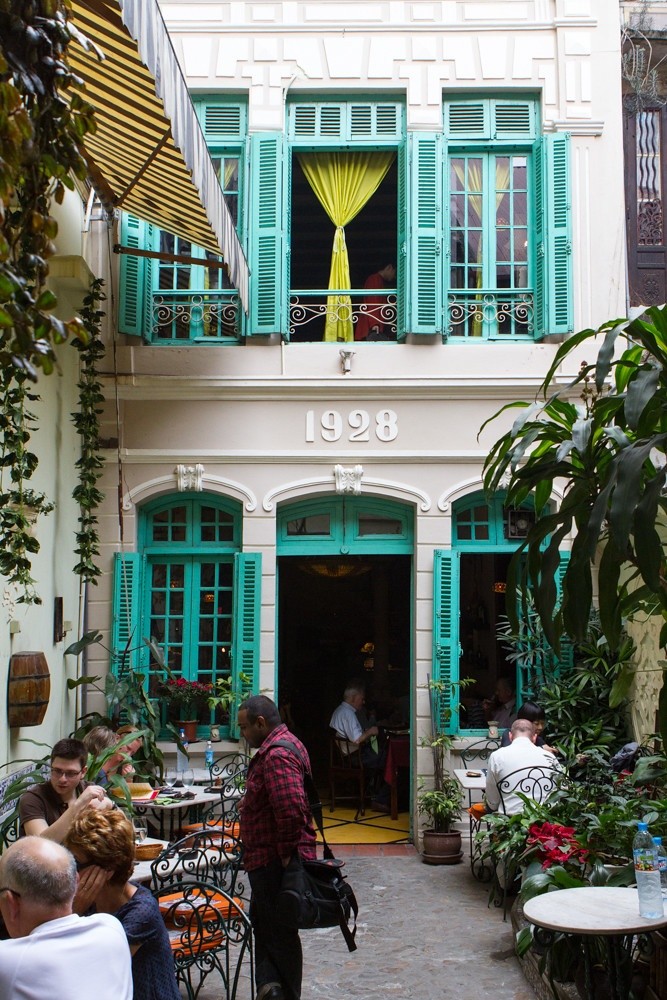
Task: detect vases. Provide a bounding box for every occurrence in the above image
[174,718,199,743]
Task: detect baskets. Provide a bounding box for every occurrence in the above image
[135,843,163,861]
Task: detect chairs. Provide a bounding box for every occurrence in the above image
[145,748,260,1000]
[325,723,366,817]
[495,766,571,921]
[458,736,503,878]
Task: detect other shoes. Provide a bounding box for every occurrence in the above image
[372,801,391,814]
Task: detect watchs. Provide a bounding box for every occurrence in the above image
[112,801,117,810]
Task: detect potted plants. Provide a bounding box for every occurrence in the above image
[415,666,478,865]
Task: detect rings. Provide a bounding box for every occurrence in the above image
[83,887,88,892]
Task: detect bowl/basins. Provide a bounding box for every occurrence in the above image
[134,844,164,861]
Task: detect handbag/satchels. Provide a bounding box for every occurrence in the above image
[280,859,359,953]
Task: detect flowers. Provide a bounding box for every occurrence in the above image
[152,671,212,720]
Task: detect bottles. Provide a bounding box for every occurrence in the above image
[653,837,667,898]
[176,729,189,771]
[205,740,214,770]
[632,823,664,919]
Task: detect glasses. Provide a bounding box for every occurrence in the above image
[50,766,83,778]
[76,860,99,872]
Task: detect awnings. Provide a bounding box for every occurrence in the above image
[53,0,251,316]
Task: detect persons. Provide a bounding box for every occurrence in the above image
[482,676,516,729]
[0,837,133,1000]
[81,726,124,785]
[63,804,182,1000]
[19,739,124,845]
[330,681,392,813]
[238,696,317,1000]
[106,725,143,783]
[500,700,558,754]
[355,258,397,341]
[485,719,566,907]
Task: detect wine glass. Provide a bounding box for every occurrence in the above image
[164,767,177,794]
[182,769,194,791]
[132,816,148,846]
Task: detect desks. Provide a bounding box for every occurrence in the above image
[128,830,236,883]
[153,764,232,838]
[451,766,488,881]
[520,883,667,1000]
[120,777,248,840]
[375,720,409,820]
